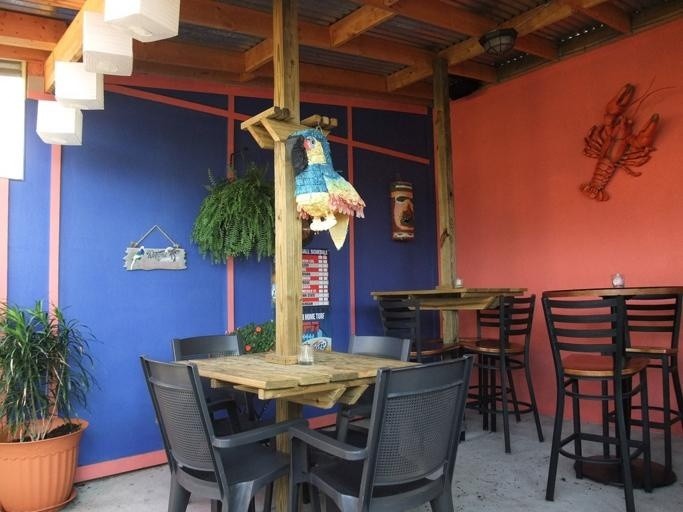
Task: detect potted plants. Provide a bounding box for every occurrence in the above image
[191,151,314,262]
[0,301,96,512]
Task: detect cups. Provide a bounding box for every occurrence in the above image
[453,277,464,289]
[610,274,625,289]
[298,343,314,365]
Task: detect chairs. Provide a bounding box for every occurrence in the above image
[371,287,544,454]
[540,287,682,511]
[138,334,474,512]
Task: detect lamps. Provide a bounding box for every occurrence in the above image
[37,0,183,145]
[481,23,518,59]
